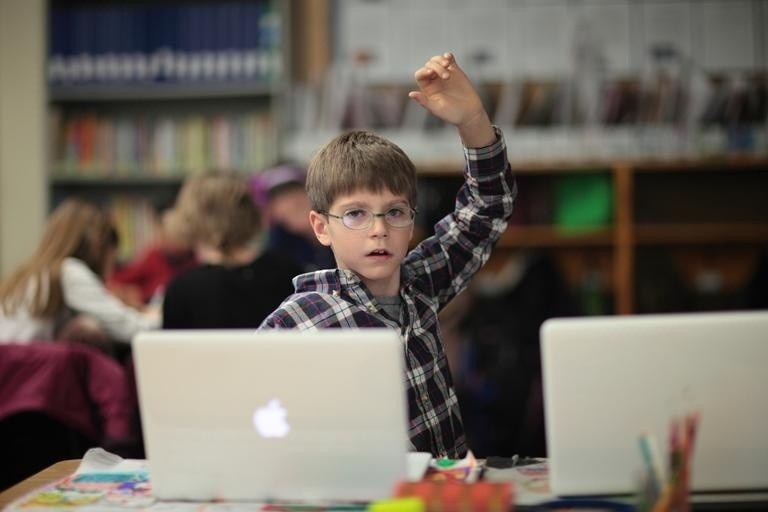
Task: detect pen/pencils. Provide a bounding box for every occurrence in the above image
[634,414,699,510]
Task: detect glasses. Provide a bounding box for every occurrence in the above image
[322,205,417,230]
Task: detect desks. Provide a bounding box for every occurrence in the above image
[0,459,765,512]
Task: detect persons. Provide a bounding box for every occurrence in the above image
[252,161,335,270]
[253,52,520,460]
[103,180,193,314]
[155,163,290,331]
[0,194,166,351]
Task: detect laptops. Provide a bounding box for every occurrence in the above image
[132,328,432,505]
[539,310,768,497]
